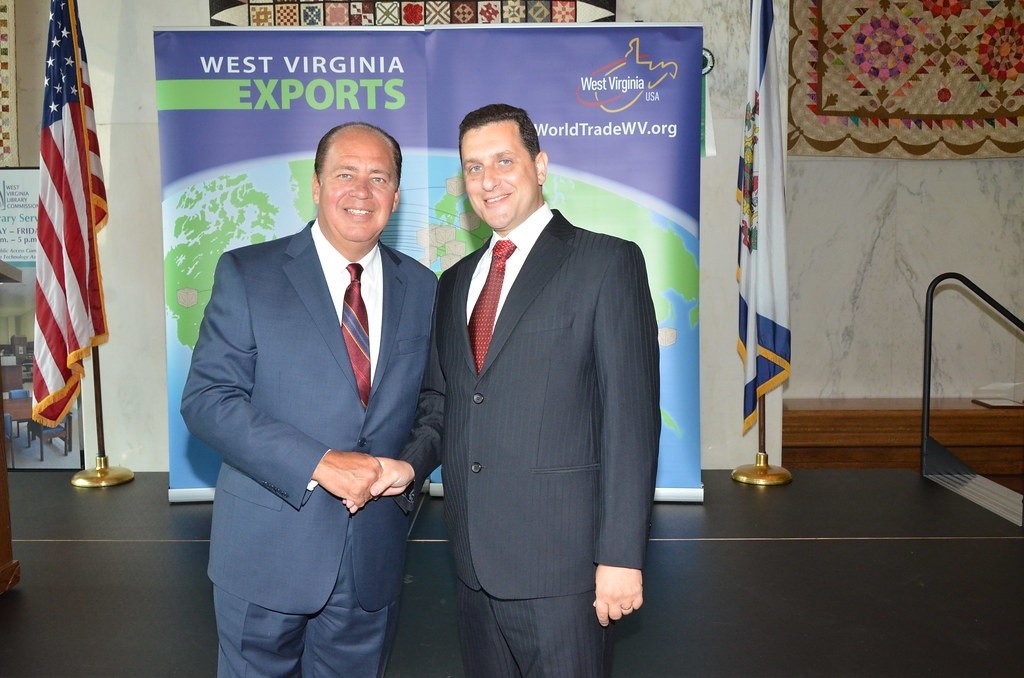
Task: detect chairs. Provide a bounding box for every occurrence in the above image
[27,412,69,461]
[8,389,30,438]
[4,413,16,468]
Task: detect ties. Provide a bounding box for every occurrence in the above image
[467,240,516,376]
[340,263,372,411]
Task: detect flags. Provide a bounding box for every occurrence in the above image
[736,0,791,438]
[32,0,110,428]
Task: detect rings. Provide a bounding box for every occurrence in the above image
[620,605,632,610]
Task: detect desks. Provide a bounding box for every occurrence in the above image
[3,397,73,452]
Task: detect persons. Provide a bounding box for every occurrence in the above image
[180,121,439,678]
[343,104,661,678]
